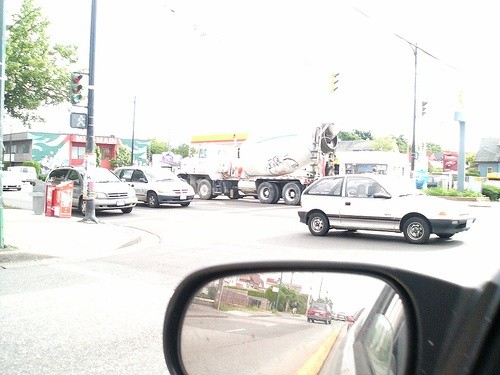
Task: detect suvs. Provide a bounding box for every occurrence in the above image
[307,303,332,325]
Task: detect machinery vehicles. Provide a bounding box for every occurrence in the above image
[176,121,342,206]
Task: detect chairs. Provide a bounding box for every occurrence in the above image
[357,184,367,197]
[368,185,372,195]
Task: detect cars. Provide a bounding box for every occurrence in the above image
[112,166,196,209]
[0,165,37,191]
[297,172,476,245]
[332,310,354,322]
[45,165,138,216]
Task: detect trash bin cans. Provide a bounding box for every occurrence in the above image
[44,178,74,218]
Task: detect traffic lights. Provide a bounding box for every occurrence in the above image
[69,112,88,129]
[330,72,339,93]
[421,100,428,116]
[70,74,83,105]
